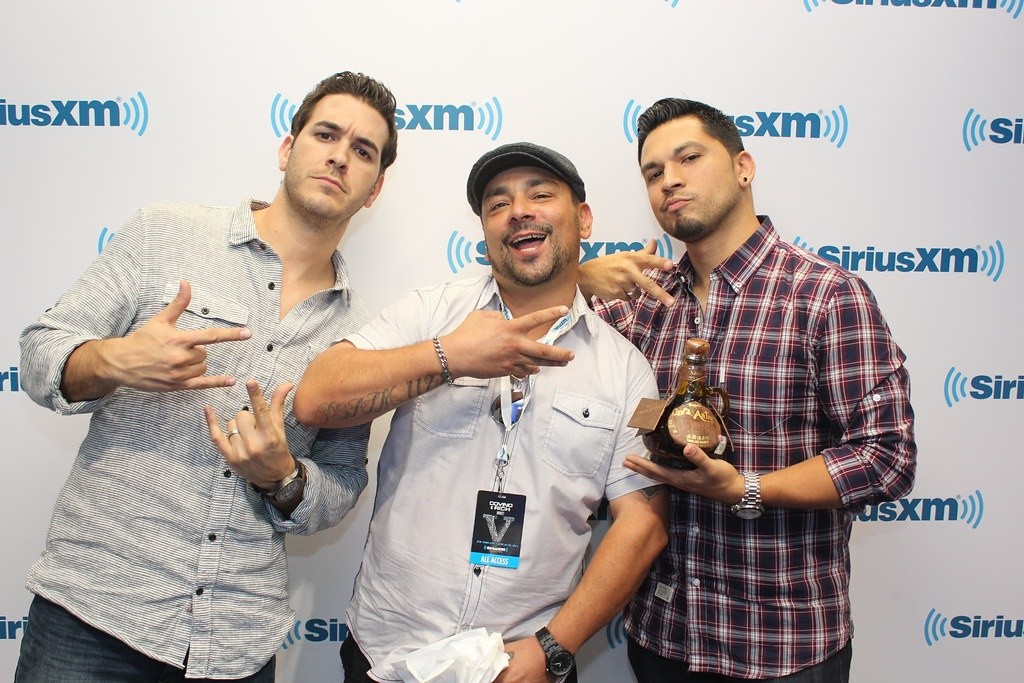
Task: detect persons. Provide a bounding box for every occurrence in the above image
[297,141,669,682]
[575,96,918,683]
[17,71,399,683]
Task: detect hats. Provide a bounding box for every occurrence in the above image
[466,141,585,216]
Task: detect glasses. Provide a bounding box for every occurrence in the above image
[493,374,526,430]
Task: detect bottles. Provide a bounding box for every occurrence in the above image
[643,337,729,461]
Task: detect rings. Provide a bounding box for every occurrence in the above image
[626,286,638,296]
[227,428,238,438]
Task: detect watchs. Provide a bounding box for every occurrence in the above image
[730,470,766,521]
[250,451,304,504]
[535,626,575,677]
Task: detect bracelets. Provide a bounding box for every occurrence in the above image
[433,336,454,387]
